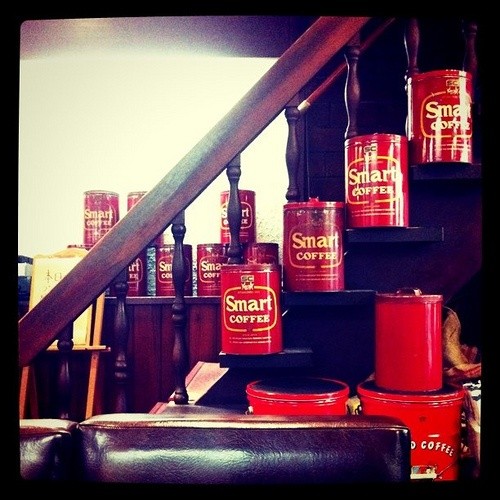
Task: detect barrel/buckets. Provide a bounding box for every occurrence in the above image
[374,288,446,392]
[245,377,349,417]
[357,380,467,483]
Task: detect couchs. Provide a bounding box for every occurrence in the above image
[20,413,411,480]
